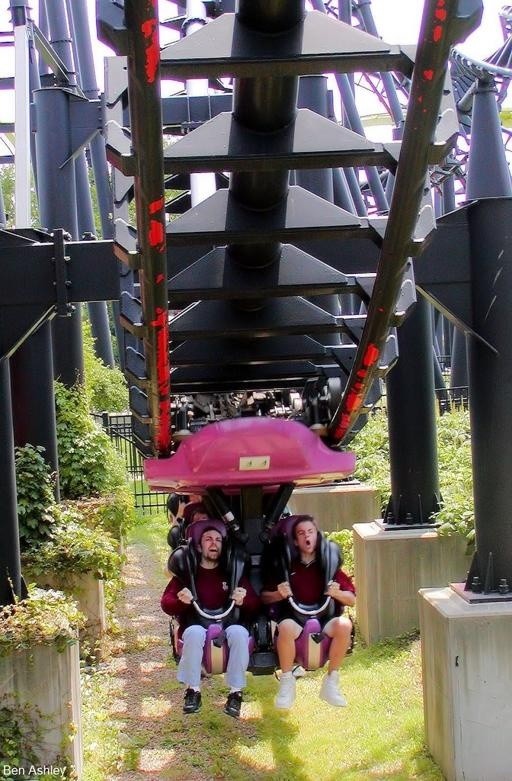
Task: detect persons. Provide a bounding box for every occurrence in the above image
[158,524,260,718]
[166,490,203,516]
[167,498,214,552]
[257,514,359,711]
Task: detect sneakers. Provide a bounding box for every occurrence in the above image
[319,670,349,707]
[223,691,244,720]
[181,688,202,715]
[275,675,297,710]
